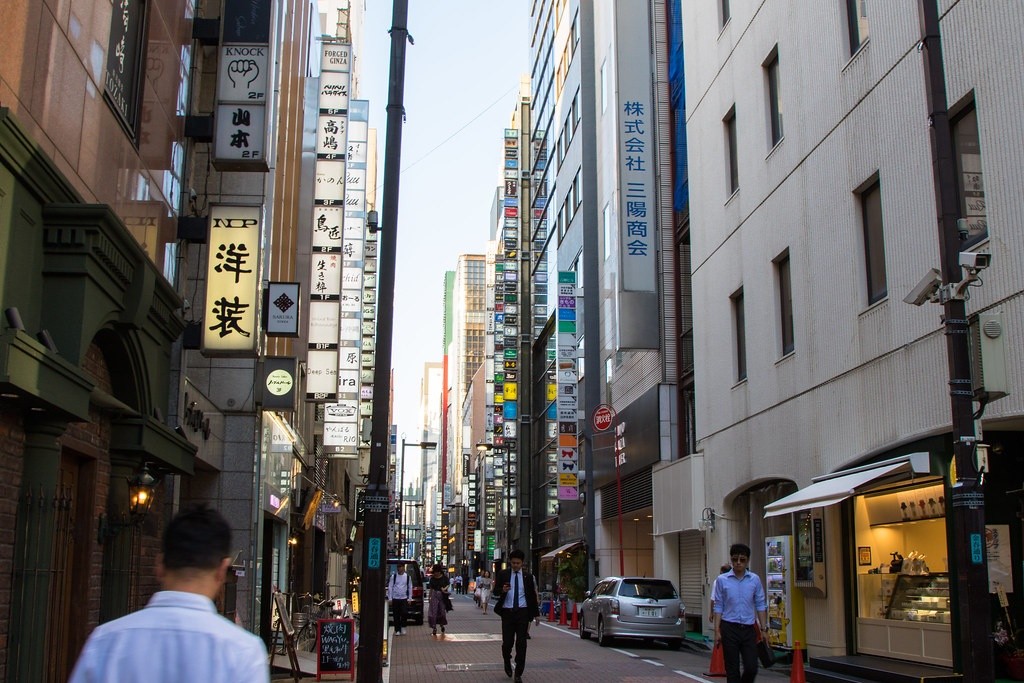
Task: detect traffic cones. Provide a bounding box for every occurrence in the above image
[702,637,727,677]
[568,604,582,630]
[545,599,558,622]
[556,601,569,626]
[788,638,807,683]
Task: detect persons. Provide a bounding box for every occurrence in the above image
[428,564,450,635]
[714,544,770,683]
[709,564,732,624]
[388,563,412,636]
[493,550,540,683]
[448,570,538,639]
[65,507,272,683]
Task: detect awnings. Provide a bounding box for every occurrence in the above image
[762,454,914,519]
[540,541,583,562]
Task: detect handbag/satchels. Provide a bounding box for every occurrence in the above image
[474,587,481,598]
[756,640,776,668]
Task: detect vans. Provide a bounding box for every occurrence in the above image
[387,558,430,625]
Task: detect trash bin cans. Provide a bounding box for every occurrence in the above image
[539,600,556,613]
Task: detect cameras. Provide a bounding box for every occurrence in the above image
[958,252,992,268]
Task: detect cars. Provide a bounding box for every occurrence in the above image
[577,576,691,650]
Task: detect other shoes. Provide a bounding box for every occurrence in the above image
[483,612,486,615]
[514,674,522,683]
[504,659,512,677]
[440,624,445,632]
[433,627,437,635]
[526,633,530,638]
[395,631,401,635]
[401,626,406,634]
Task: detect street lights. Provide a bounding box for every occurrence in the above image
[446,503,466,596]
[404,503,425,559]
[406,527,422,559]
[396,439,438,558]
[476,443,512,570]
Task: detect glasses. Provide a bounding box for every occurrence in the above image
[732,557,748,563]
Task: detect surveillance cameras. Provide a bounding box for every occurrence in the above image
[901,266,943,307]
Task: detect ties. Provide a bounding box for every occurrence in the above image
[513,571,519,613]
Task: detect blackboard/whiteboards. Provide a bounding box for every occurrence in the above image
[316,619,356,674]
[274,592,297,636]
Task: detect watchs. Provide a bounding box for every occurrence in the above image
[760,627,769,632]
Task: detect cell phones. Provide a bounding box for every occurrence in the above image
[504,582,510,587]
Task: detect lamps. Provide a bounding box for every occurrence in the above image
[579,492,586,505]
[554,503,560,515]
[96,460,163,546]
[698,507,715,533]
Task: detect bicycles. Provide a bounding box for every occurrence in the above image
[296,594,361,652]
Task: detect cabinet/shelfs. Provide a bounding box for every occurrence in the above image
[885,571,951,624]
